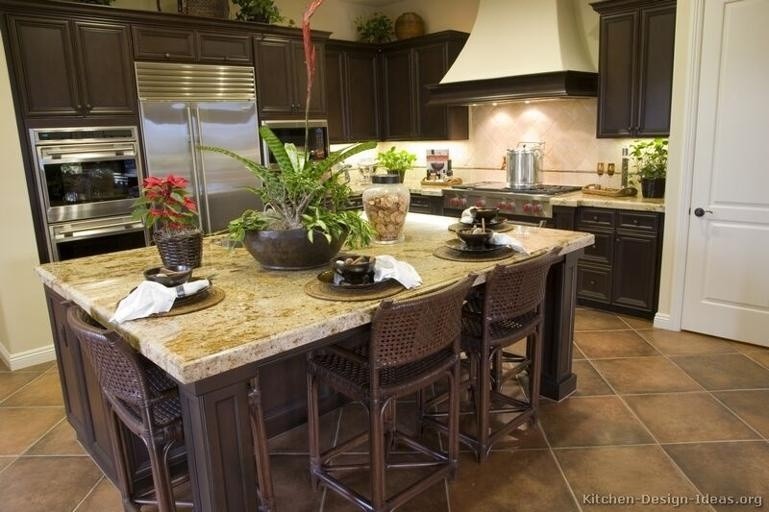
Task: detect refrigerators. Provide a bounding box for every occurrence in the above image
[138,97,266,241]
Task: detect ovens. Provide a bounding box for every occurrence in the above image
[27,125,150,263]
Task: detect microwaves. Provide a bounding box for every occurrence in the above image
[260,118,330,172]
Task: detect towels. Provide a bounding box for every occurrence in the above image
[460,205,478,225]
[488,230,530,259]
[108,281,178,325]
[374,251,425,290]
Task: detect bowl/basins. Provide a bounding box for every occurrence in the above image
[143,264,193,287]
[470,207,499,222]
[456,228,493,247]
[331,255,376,280]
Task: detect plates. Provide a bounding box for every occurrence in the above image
[166,277,212,307]
[459,217,503,229]
[317,270,390,292]
[445,238,507,255]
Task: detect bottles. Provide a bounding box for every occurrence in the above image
[446,160,453,177]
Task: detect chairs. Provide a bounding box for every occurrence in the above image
[302,272,477,511]
[417,240,565,462]
[59,309,186,511]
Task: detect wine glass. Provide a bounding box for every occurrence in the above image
[607,163,615,188]
[596,162,604,189]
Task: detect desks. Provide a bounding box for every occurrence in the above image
[34,210,597,511]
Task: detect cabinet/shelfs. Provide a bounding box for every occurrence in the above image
[332,194,363,213]
[573,207,617,304]
[613,210,659,315]
[250,23,328,120]
[43,287,152,496]
[410,194,442,214]
[133,9,252,64]
[0,0,133,123]
[593,0,678,139]
[376,30,470,141]
[324,41,375,144]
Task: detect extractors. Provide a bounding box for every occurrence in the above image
[423,72,598,107]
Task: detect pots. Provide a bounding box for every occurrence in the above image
[504,145,544,188]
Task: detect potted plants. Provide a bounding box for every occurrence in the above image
[132,174,205,272]
[352,14,394,44]
[380,146,416,184]
[633,138,669,198]
[194,0,376,271]
[231,1,287,25]
